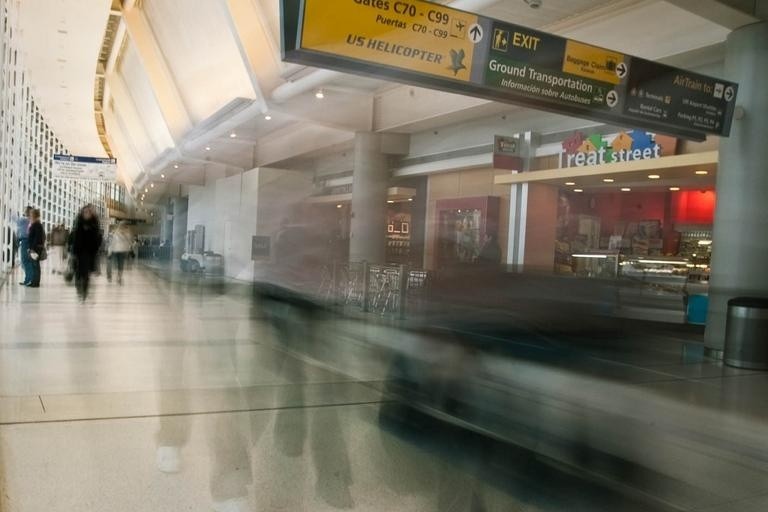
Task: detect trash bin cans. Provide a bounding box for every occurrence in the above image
[724,297,768,370]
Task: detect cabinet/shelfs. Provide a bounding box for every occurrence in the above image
[614,254,691,294]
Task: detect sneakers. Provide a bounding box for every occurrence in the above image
[19,281,40,287]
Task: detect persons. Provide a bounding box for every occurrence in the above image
[15,202,142,303]
[474,230,502,266]
